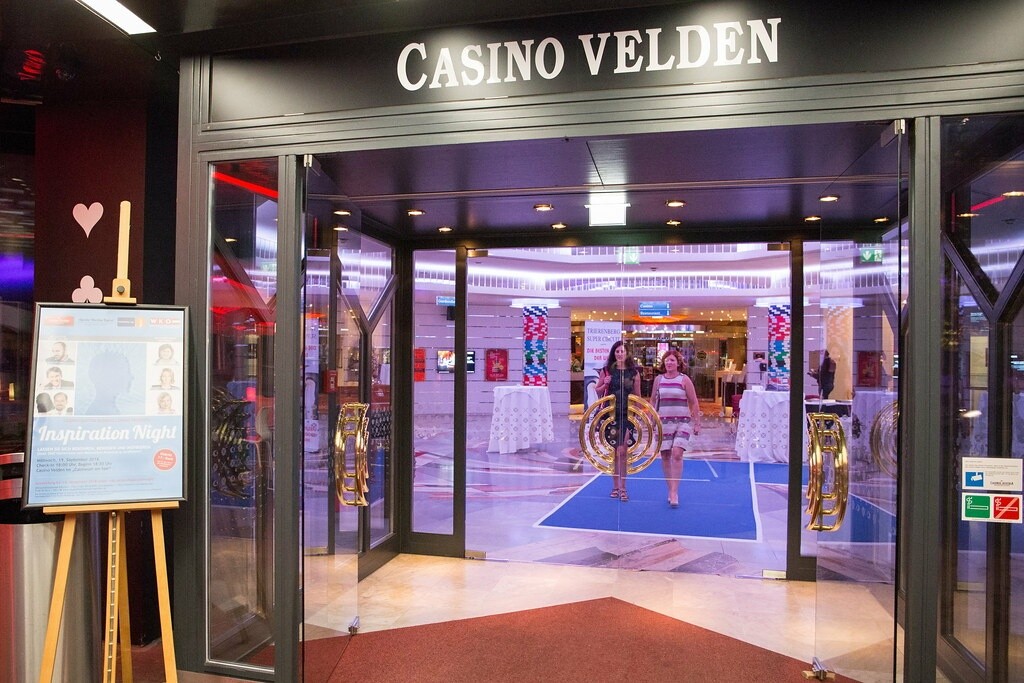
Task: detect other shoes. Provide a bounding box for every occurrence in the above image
[668,491,679,506]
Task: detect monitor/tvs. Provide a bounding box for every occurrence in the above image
[437,350,475,373]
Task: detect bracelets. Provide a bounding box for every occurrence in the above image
[695,424,700,426]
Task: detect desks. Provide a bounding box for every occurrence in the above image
[852,390,899,460]
[486,385,554,454]
[734,389,810,464]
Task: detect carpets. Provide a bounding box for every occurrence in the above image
[246,596,864,683]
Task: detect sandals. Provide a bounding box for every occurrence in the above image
[610,487,628,502]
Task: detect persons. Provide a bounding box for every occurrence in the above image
[150,369,178,390]
[648,349,700,506]
[156,393,174,414]
[810,351,836,400]
[45,342,74,364]
[43,366,73,389]
[595,341,641,501]
[153,345,179,366]
[47,393,73,415]
